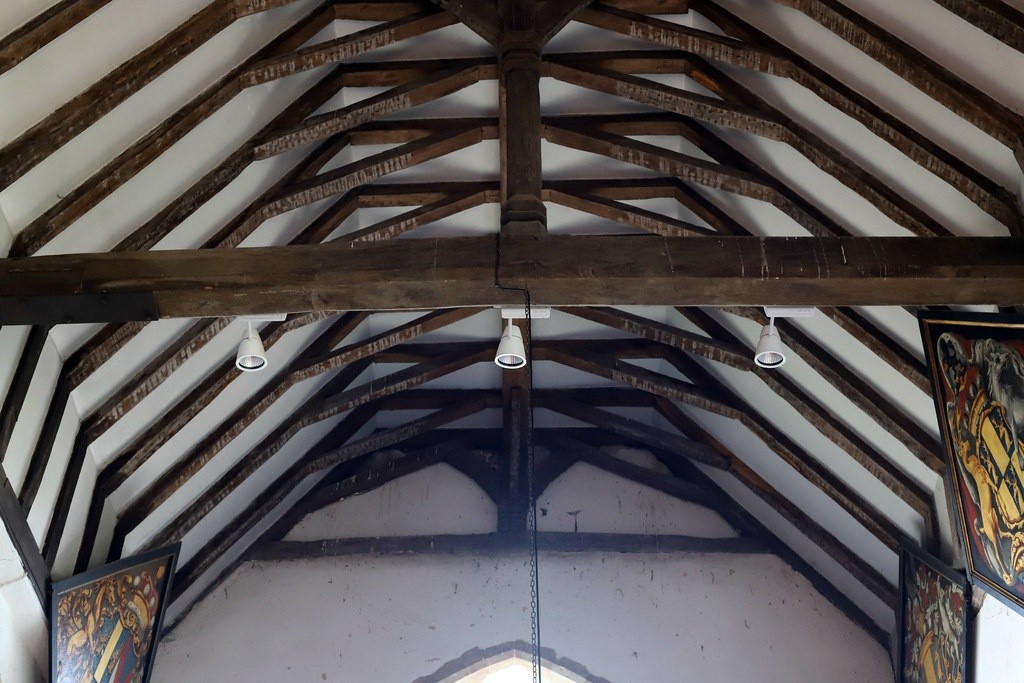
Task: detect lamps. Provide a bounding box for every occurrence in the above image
[754,317,786,369]
[494,319,526,369]
[237,320,267,372]
